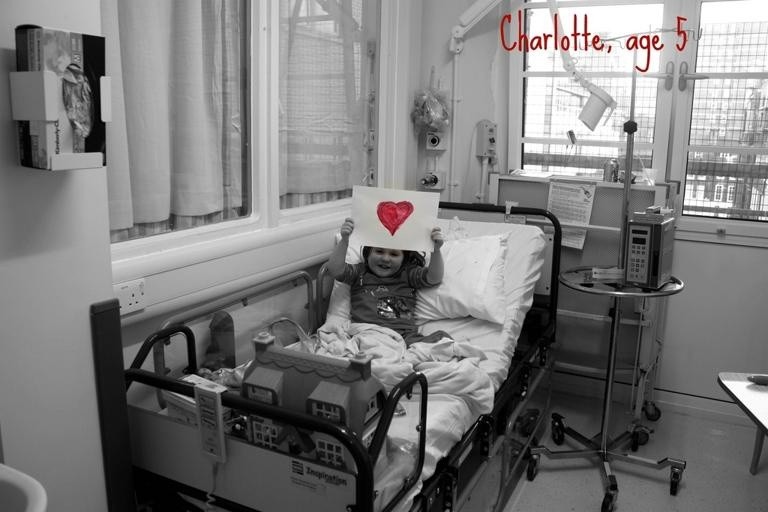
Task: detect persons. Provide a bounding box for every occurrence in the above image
[328,217,444,347]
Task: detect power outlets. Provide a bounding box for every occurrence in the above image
[106,278,149,314]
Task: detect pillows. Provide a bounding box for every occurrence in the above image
[326,232,511,325]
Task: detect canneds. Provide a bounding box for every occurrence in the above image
[602,158,619,183]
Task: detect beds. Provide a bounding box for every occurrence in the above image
[89,201,562,512]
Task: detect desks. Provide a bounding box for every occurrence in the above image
[717,372,768,475]
